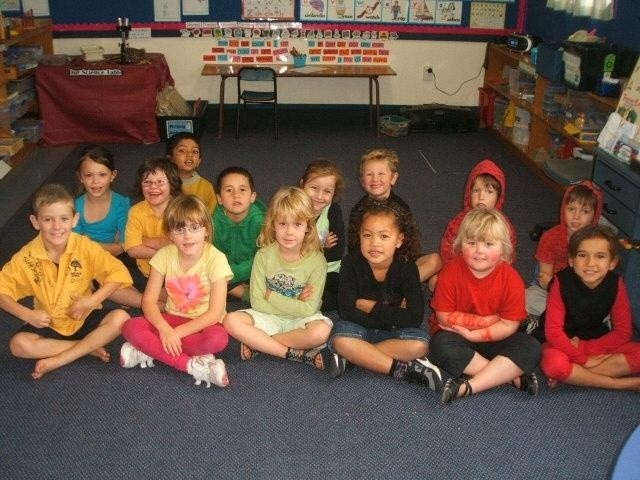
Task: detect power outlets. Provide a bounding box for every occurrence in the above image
[423,66,433,80]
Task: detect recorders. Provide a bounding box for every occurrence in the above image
[400,103,479,134]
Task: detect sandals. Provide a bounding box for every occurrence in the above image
[285,342,330,370]
[241,342,252,362]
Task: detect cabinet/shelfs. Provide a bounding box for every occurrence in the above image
[34,52,174,144]
[591,145,639,328]
[0,18,54,168]
[482,43,622,199]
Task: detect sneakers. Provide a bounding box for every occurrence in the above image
[188,353,229,388]
[407,355,444,392]
[120,342,156,369]
[330,354,346,379]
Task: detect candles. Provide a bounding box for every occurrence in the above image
[125,17,129,26]
[118,18,122,28]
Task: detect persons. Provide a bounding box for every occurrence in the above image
[222,186,334,371]
[0,183,134,380]
[209,166,269,303]
[327,198,445,395]
[428,160,518,293]
[92,157,184,309]
[165,132,217,215]
[299,157,345,312]
[71,146,131,258]
[524,179,614,336]
[348,148,443,284]
[118,193,234,388]
[428,208,543,404]
[538,225,640,393]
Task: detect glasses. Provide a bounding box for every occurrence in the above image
[173,223,202,235]
[141,179,171,187]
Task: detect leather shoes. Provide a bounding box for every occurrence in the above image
[512,373,538,396]
[442,376,472,403]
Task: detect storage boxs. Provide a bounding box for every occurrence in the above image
[561,45,606,92]
[12,119,44,144]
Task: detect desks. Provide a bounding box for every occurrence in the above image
[200,63,397,139]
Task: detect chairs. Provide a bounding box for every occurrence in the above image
[236,66,278,139]
